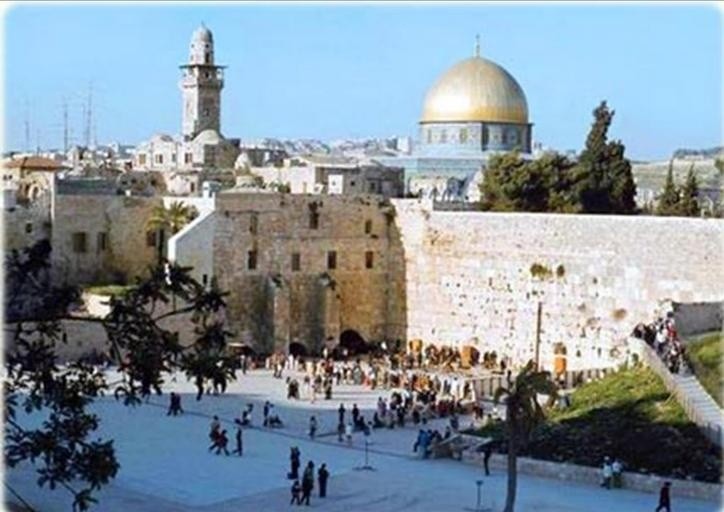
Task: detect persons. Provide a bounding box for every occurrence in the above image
[634,311,682,374]
[270,337,505,440]
[168,392,181,416]
[601,456,612,489]
[208,415,242,456]
[288,447,329,506]
[611,457,624,487]
[413,425,451,456]
[235,351,269,370]
[654,481,672,512]
[236,400,282,426]
